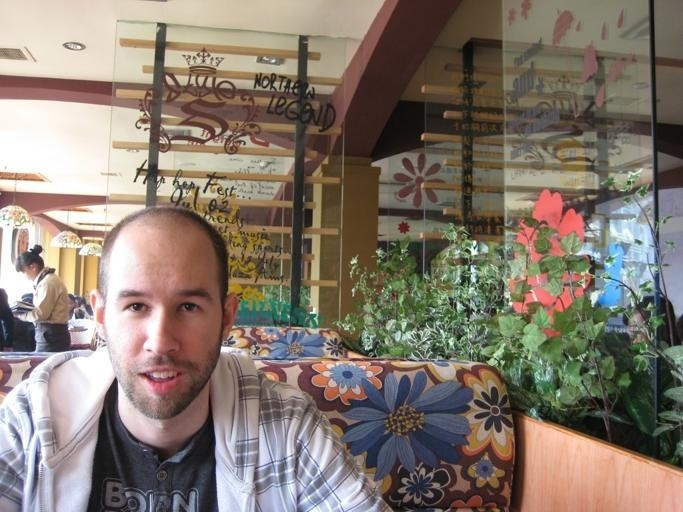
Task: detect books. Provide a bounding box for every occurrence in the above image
[9,300,35,313]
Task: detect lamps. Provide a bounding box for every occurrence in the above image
[0,174,36,229]
[49,209,102,257]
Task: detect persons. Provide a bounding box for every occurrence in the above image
[0,202,396,512]
[0,287,14,352]
[68,293,93,320]
[21,293,33,301]
[13,244,72,352]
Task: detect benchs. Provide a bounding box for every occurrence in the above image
[0,324,515,512]
